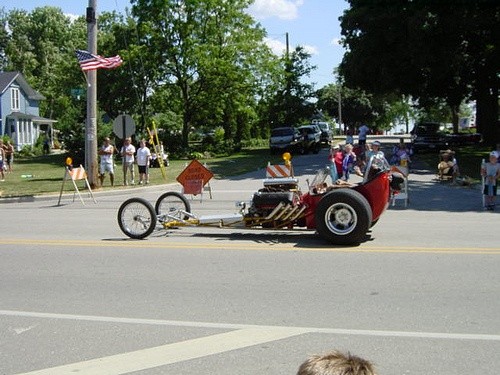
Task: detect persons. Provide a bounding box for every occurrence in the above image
[346,124,354,144]
[296,351,376,375]
[98,137,114,186]
[136,141,150,184]
[480,150,500,211]
[298,128,309,155]
[358,120,370,145]
[328,141,409,196]
[0,139,15,181]
[438,153,458,184]
[119,137,136,186]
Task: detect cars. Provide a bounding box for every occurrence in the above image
[412,123,445,152]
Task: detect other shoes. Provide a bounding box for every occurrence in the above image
[138,182,142,185]
[145,182,149,184]
[124,183,128,186]
[131,183,134,185]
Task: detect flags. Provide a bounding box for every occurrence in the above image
[75,49,124,72]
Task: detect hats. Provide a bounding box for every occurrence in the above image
[372,141,381,146]
[332,144,341,149]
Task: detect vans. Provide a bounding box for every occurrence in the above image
[270,125,332,155]
